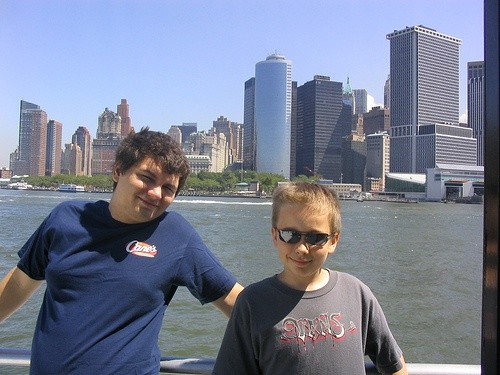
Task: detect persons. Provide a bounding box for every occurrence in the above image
[210,182,408,375]
[0,126,244,375]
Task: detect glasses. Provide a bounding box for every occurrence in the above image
[274,226,333,245]
